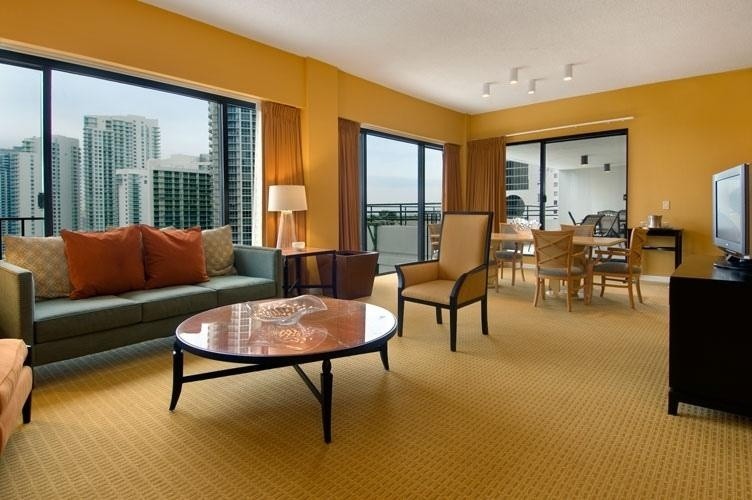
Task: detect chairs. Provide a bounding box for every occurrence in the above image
[394,210,494,352]
[428,223,649,312]
[568,210,627,250]
[0,338,35,459]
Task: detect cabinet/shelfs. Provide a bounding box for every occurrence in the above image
[667,253,752,422]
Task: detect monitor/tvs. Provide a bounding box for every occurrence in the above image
[711,162,752,270]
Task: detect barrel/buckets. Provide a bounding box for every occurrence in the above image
[648,215,662,228]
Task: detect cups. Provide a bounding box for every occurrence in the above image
[292,242,305,251]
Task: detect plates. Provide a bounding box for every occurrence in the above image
[247,321,328,352]
[244,295,329,325]
[510,216,542,239]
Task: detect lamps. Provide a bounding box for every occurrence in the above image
[267,185,308,252]
[482,63,573,98]
[581,139,610,172]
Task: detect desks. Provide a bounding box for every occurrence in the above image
[628,227,683,283]
[281,247,338,299]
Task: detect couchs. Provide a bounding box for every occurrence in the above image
[0,243,284,390]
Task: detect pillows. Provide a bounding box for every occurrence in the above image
[4,224,238,300]
